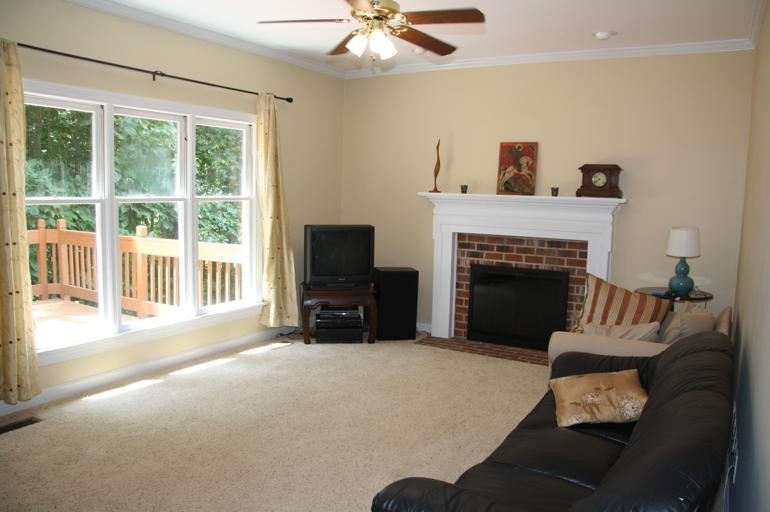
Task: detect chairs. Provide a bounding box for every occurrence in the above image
[547,305,732,389]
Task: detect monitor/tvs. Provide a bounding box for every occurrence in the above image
[303,224,375,290]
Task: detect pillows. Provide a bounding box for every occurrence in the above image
[548,368,649,428]
[569,273,714,344]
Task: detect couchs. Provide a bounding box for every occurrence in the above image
[371,331,736,512]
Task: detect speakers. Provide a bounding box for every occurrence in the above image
[376,266,419,341]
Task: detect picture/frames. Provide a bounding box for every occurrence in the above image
[495,142,538,196]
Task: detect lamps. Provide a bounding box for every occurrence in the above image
[594,32,612,39]
[665,227,702,296]
[346,21,398,62]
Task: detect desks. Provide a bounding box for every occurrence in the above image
[302,288,378,343]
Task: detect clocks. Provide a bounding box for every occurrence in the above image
[575,163,624,199]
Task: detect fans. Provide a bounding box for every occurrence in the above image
[255,0,485,55]
